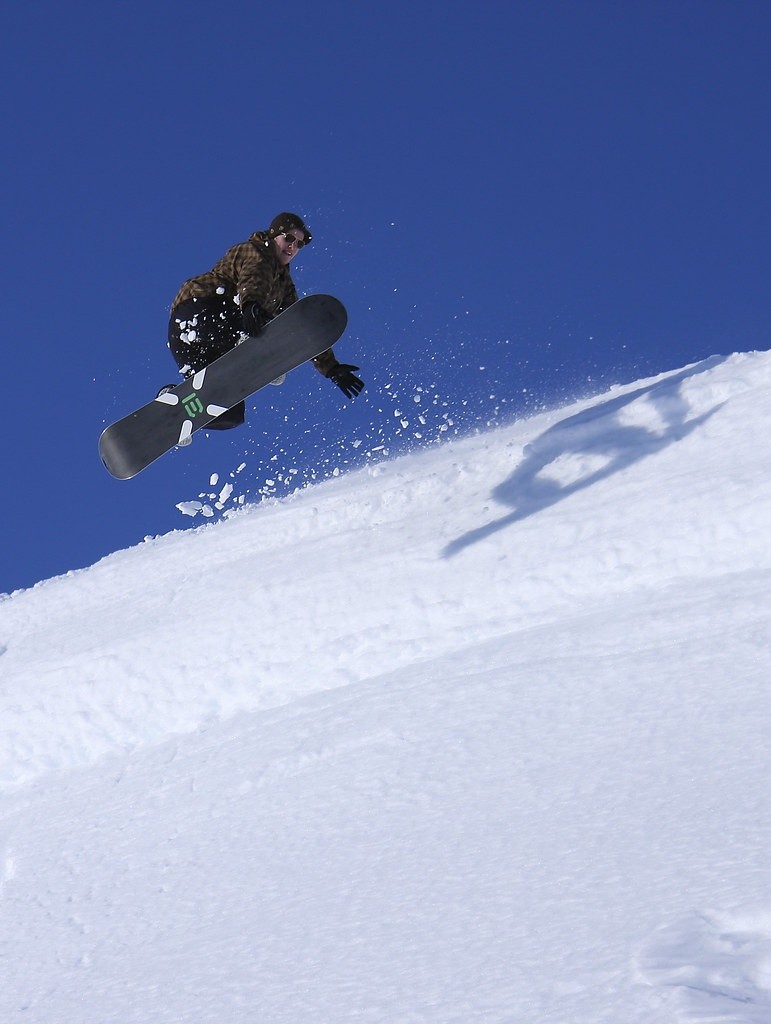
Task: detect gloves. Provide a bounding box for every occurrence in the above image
[241,300,275,330]
[327,363,364,399]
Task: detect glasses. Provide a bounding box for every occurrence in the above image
[281,232,305,249]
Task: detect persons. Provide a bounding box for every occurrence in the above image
[156,211,363,431]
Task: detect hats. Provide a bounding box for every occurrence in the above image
[269,213,312,245]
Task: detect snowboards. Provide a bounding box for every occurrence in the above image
[98,292,350,481]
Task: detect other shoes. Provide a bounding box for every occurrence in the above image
[155,384,177,398]
